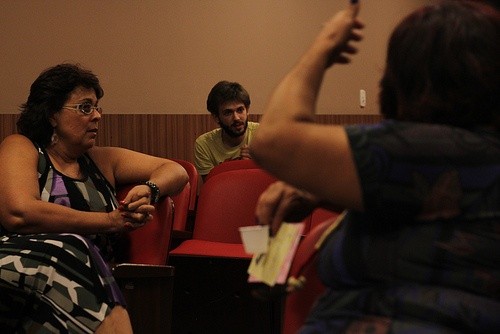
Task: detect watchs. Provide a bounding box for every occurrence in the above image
[145,181,160,204]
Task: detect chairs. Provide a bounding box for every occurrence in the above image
[116,158,340,334]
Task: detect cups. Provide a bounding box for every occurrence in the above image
[238,225,270,254]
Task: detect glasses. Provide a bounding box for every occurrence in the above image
[60,103,103,114]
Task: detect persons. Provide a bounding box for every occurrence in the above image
[250,0,500,334]
[0,63,189,334]
[194,81,261,184]
